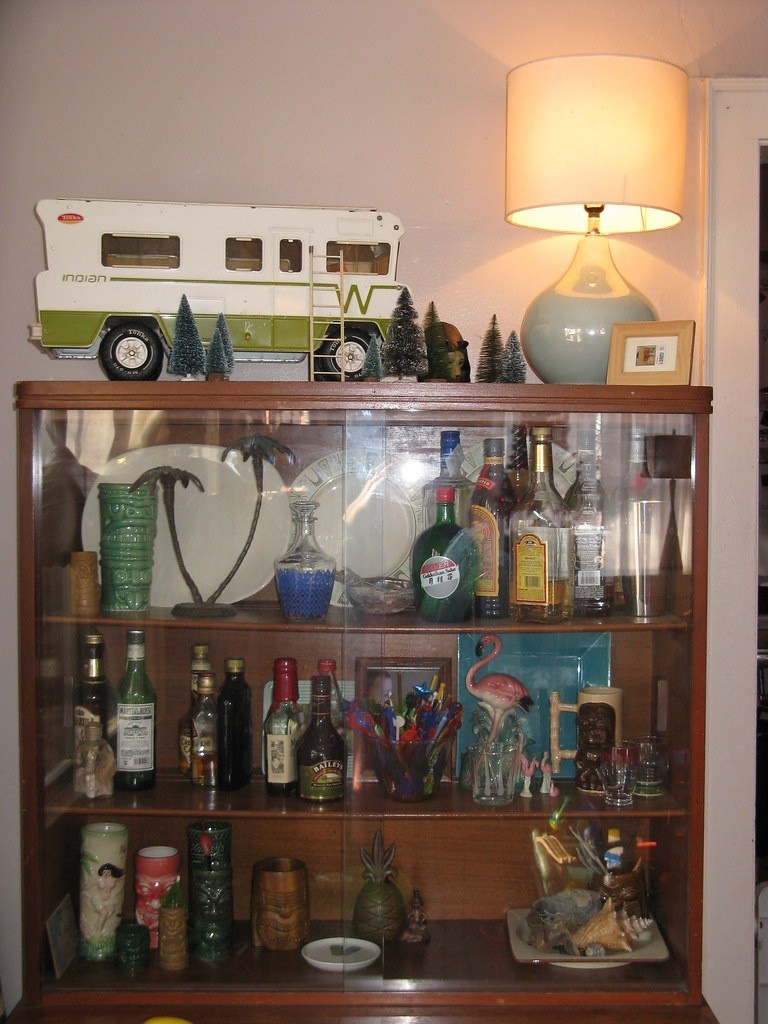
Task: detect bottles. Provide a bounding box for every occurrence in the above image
[73,633,345,803]
[273,428,671,623]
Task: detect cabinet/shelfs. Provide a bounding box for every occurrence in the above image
[6,370,713,1008]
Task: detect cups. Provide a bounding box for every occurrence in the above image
[116,922,150,971]
[600,735,673,805]
[468,745,517,805]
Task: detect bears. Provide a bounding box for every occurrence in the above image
[417,322,471,382]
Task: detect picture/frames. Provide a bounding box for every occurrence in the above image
[607,319,696,384]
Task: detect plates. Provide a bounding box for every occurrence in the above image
[506,906,669,969]
[301,937,381,972]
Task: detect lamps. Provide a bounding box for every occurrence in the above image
[506,54,688,383]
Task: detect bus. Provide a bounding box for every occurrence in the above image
[27,197,406,382]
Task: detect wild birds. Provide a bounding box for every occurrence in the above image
[465,633,536,783]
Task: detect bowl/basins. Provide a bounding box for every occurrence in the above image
[363,728,456,802]
[346,578,416,613]
